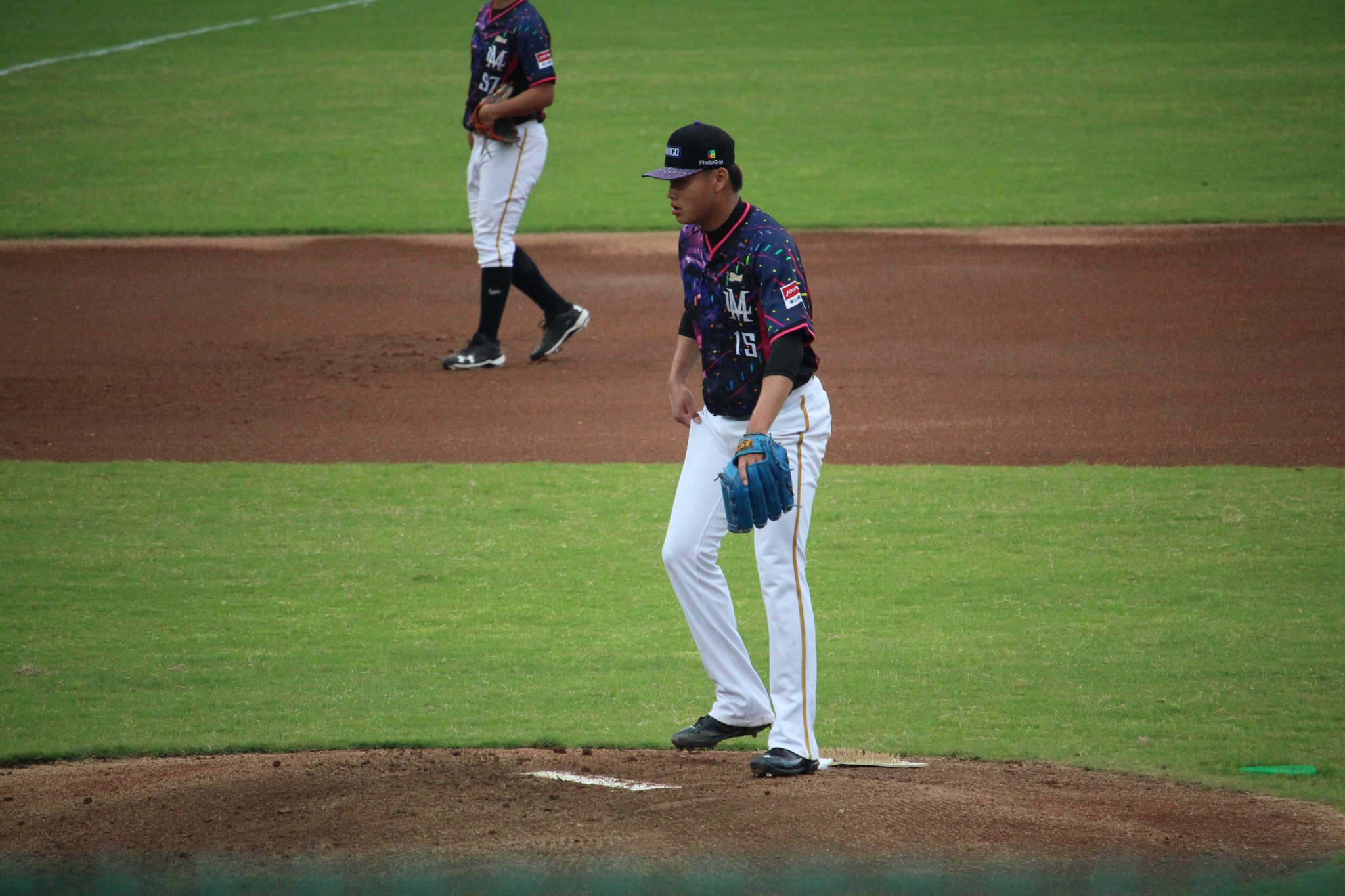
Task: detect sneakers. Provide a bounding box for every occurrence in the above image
[749,748,819,776]
[671,714,772,749]
[530,302,590,361]
[442,336,505,369]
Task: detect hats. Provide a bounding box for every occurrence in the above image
[642,122,735,180]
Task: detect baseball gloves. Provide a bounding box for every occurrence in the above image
[720,433,794,534]
[471,81,521,145]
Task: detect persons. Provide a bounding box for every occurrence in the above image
[641,122,833,779]
[440,2,591,371]
